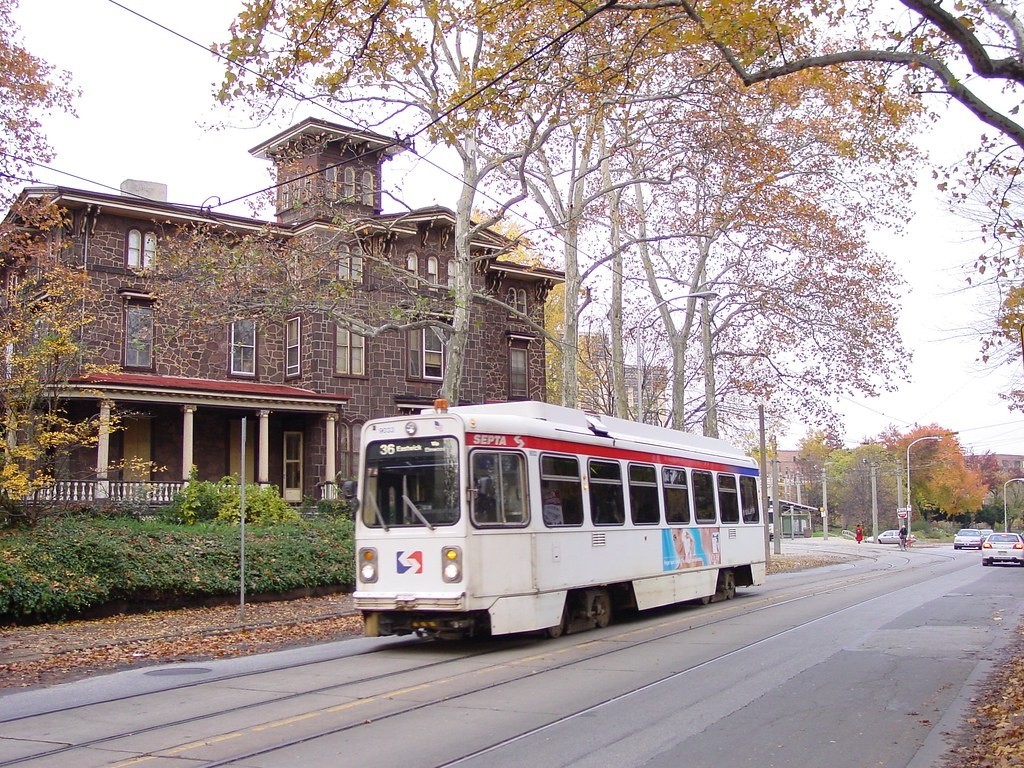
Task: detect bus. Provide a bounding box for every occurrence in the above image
[354,396,770,640]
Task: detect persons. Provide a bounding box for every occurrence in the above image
[462,478,492,522]
[898,525,907,547]
[855,524,863,542]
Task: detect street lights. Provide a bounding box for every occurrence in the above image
[906,436,944,547]
[1003,477,1024,532]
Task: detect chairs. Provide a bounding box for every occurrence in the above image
[543,489,563,526]
[637,501,659,523]
[741,501,753,521]
[599,501,620,522]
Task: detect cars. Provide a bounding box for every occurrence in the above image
[953,528,982,550]
[982,533,1024,569]
[865,529,917,544]
[980,528,995,543]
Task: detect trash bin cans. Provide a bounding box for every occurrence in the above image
[803,527,812,538]
